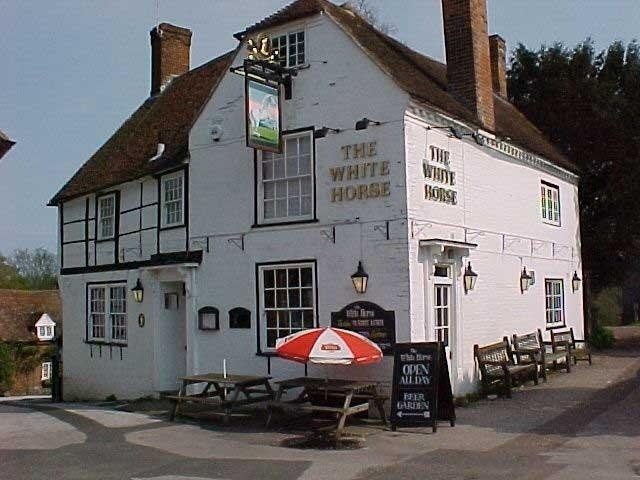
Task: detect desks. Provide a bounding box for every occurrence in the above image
[262,375,390,438]
[174,371,273,427]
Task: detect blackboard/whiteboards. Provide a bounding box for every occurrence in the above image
[391,342,440,427]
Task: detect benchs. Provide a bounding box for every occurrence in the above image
[550,328,592,365]
[161,393,243,426]
[263,400,349,438]
[513,328,571,383]
[221,387,287,400]
[474,336,539,399]
[308,390,390,424]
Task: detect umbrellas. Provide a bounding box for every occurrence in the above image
[276,325,384,401]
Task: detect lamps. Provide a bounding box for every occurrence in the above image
[431,127,462,139]
[313,127,340,138]
[572,270,581,293]
[520,266,532,294]
[129,276,144,303]
[356,118,380,130]
[462,134,483,146]
[464,261,478,291]
[350,261,368,294]
